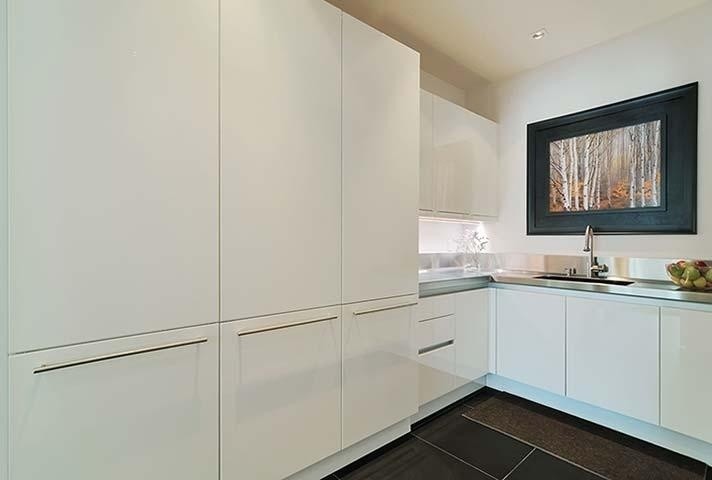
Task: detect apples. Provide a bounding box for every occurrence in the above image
[677,261,707,271]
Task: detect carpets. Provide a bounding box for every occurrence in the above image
[462,395,709,480]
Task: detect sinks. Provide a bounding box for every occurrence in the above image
[533,275,635,288]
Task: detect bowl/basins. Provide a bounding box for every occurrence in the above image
[664,262,712,291]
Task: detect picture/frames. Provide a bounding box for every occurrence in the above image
[526,82,698,235]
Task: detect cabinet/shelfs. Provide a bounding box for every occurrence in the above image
[485,287,495,389]
[566,278,660,446]
[660,286,710,464]
[411,288,487,430]
[340,294,411,470]
[485,287,712,467]
[221,304,341,480]
[4,1,219,355]
[419,87,500,222]
[341,10,419,305]
[4,323,221,479]
[220,1,340,322]
[496,272,567,413]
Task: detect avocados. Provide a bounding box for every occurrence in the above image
[667,263,683,276]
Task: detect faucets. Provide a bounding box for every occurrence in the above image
[583,225,594,264]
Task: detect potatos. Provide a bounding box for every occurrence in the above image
[672,277,707,288]
